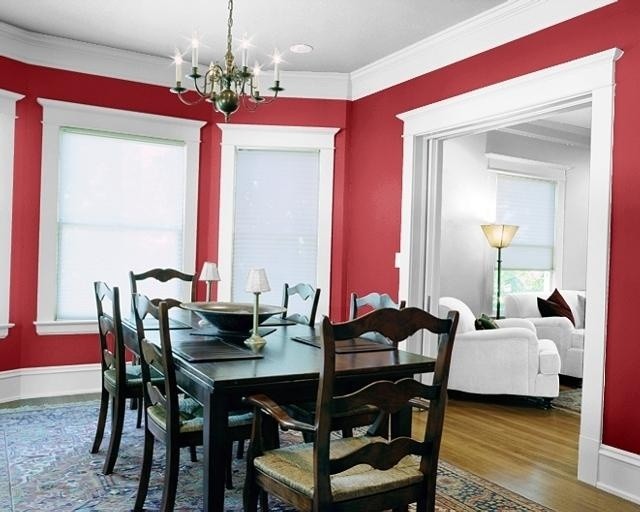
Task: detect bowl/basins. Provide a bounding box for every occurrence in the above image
[179,300,289,335]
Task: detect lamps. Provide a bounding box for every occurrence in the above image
[481,224,519,318]
[169,0,284,122]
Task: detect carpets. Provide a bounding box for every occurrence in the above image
[537,386,581,415]
[0,395,555,512]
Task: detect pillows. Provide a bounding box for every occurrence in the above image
[536,288,575,327]
[475,313,500,330]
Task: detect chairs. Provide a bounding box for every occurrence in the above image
[504,291,585,389]
[437,297,560,409]
